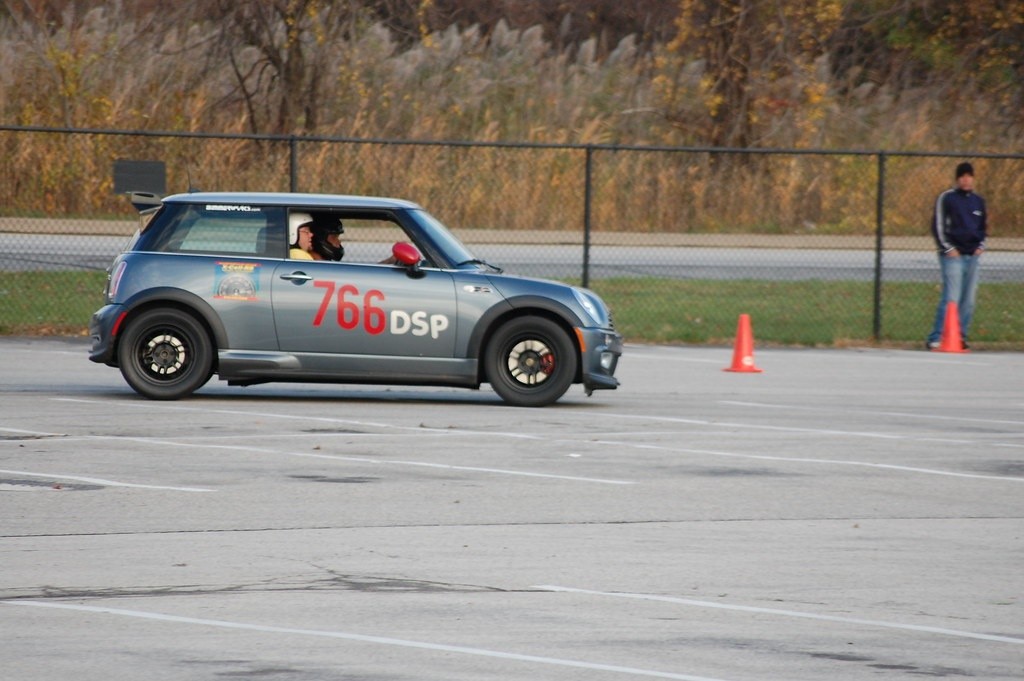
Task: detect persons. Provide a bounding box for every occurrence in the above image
[287,210,396,264]
[927,163,986,347]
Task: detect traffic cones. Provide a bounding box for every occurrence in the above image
[722,313,764,373]
[930,301,971,354]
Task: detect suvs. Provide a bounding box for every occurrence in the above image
[86,189,625,408]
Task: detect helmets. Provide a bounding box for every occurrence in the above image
[288,213,312,245]
[309,217,347,261]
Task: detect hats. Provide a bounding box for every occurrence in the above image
[955,162,974,177]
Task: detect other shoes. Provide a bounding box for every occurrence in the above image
[928,342,939,349]
[963,341,968,349]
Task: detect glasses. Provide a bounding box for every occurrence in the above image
[298,230,311,235]
